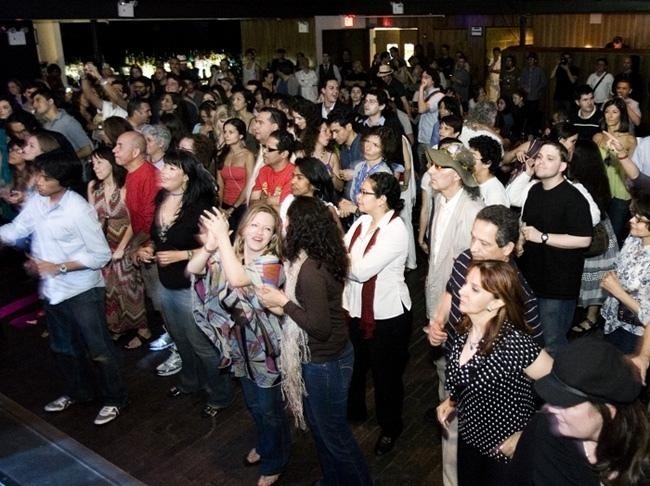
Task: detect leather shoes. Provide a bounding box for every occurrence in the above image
[373,433,395,457]
[198,404,222,420]
[242,448,260,468]
[165,384,190,401]
[256,474,281,486]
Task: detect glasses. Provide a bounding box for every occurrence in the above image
[430,162,454,169]
[262,144,282,153]
[359,188,377,196]
[635,215,650,224]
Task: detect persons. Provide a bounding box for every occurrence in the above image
[628,320,650,386]
[603,130,650,219]
[468,85,488,123]
[0,79,33,116]
[160,113,183,155]
[407,42,471,102]
[468,135,508,207]
[507,159,602,227]
[33,61,95,112]
[423,204,543,486]
[339,125,400,219]
[508,334,650,486]
[247,107,298,206]
[140,149,239,419]
[185,200,290,486]
[494,96,514,134]
[503,122,579,190]
[112,131,183,376]
[342,47,407,94]
[257,195,372,486]
[291,102,318,139]
[175,133,215,169]
[331,118,364,200]
[217,118,256,246]
[243,48,342,110]
[7,138,38,210]
[549,36,640,117]
[140,124,171,172]
[417,137,462,255]
[593,98,637,237]
[486,46,545,95]
[193,100,216,137]
[102,116,134,147]
[214,105,236,169]
[542,105,571,138]
[249,130,296,213]
[8,108,84,193]
[436,259,556,486]
[429,96,461,147]
[431,115,462,150]
[0,150,133,427]
[512,88,541,142]
[382,101,417,274]
[0,128,18,224]
[596,197,650,354]
[384,89,414,145]
[89,146,152,350]
[425,144,489,401]
[95,60,153,122]
[567,137,620,340]
[297,118,343,192]
[511,141,594,352]
[345,172,412,459]
[23,133,59,160]
[353,88,388,132]
[153,55,242,114]
[279,156,340,241]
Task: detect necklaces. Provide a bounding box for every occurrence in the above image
[468,332,480,350]
[169,193,183,196]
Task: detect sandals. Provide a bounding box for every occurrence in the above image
[124,333,152,351]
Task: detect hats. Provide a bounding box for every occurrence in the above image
[425,142,481,198]
[530,338,643,405]
[376,65,394,77]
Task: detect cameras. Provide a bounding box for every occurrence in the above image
[560,56,568,65]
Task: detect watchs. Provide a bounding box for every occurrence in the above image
[98,124,103,130]
[59,263,67,274]
[541,232,548,244]
[203,244,217,254]
[188,250,193,261]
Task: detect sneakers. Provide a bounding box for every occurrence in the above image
[155,347,182,378]
[44,395,77,415]
[148,332,174,351]
[93,403,126,426]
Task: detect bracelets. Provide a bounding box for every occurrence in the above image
[616,152,629,160]
[231,205,237,210]
